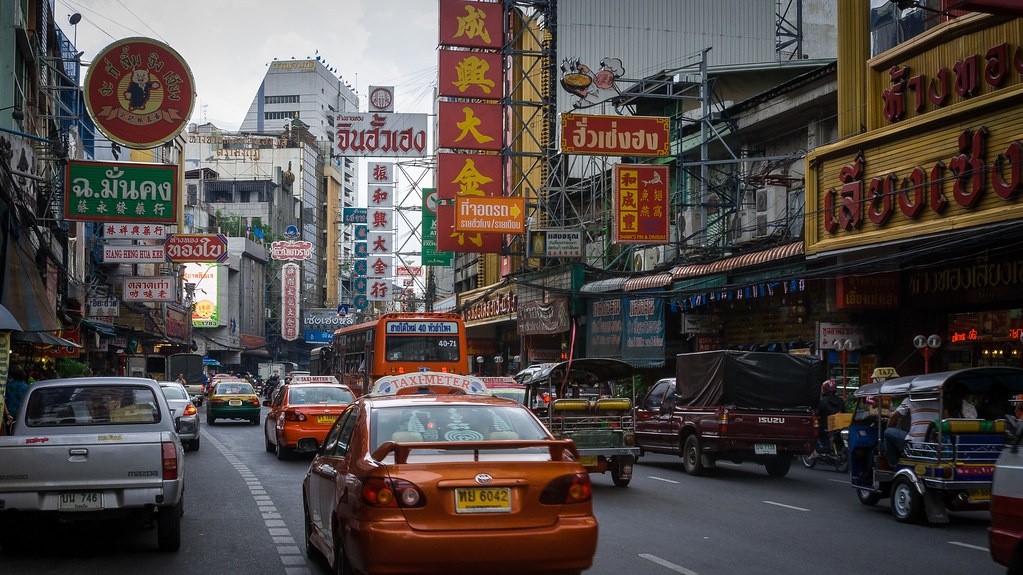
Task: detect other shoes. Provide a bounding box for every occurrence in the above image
[808,453,819,460]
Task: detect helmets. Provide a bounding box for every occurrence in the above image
[821,380,836,396]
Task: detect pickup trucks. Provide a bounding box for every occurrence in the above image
[0,376,199,555]
[625,378,821,481]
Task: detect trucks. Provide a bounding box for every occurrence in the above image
[165,352,204,407]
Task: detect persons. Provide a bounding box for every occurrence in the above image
[883,384,979,475]
[805,380,850,462]
[4,360,62,426]
[236,371,291,408]
[175,372,187,387]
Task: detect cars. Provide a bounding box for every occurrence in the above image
[987,414,1023,575]
[297,371,599,575]
[474,376,617,417]
[262,376,358,462]
[204,361,285,426]
[58,380,202,452]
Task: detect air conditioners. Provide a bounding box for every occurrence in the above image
[754,185,788,237]
[633,248,657,272]
[729,209,755,243]
[677,209,706,253]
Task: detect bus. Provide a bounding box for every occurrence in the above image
[319,311,469,401]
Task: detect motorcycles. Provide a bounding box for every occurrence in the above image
[801,412,852,474]
[513,358,642,488]
[846,365,1022,529]
